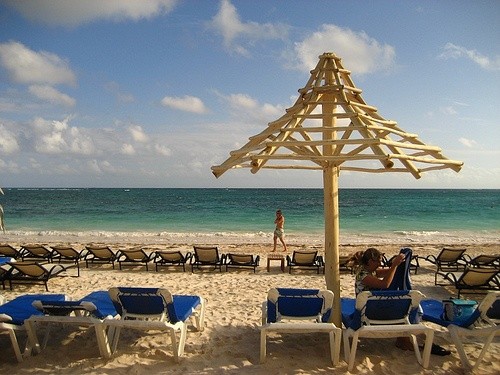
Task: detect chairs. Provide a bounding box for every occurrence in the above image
[462,253,500,267]
[51,246,86,264]
[4,261,66,290]
[117,249,153,272]
[434,269,500,298]
[417,291,500,371]
[24,289,115,357]
[341,289,435,372]
[21,242,50,257]
[154,250,191,272]
[0,293,72,365]
[189,245,224,273]
[261,287,337,365]
[382,254,420,274]
[225,253,260,274]
[286,250,321,274]
[104,287,205,360]
[84,246,119,269]
[0,244,28,258]
[340,253,355,274]
[418,247,467,272]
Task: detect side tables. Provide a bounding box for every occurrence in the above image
[266,253,284,273]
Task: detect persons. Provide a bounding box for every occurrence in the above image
[271,210,287,252]
[354,248,406,298]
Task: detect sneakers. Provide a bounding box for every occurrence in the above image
[423,342,451,356]
